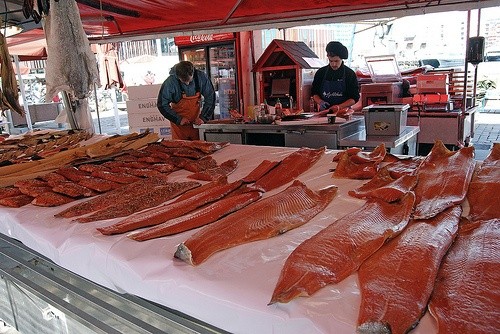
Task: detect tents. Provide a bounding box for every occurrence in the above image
[0,29,125,131]
[62,0,500,133]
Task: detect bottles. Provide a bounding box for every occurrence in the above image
[310,96,315,112]
[275,98,283,113]
[257,99,270,115]
[227,107,242,118]
[246,99,254,118]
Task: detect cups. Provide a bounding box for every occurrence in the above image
[326,114,337,123]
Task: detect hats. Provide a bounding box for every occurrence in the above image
[326,41,348,59]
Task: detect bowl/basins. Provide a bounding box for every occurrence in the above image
[258,115,276,124]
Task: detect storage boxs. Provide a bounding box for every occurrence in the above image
[412,74,452,110]
[126,84,172,140]
[362,102,410,136]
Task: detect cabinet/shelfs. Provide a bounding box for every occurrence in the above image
[193,114,362,149]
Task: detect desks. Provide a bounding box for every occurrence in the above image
[339,126,420,156]
[407,105,476,150]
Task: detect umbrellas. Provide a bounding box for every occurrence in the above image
[98,43,124,133]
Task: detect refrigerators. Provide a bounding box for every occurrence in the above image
[174,29,263,122]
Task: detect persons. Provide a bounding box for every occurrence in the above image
[311,41,360,113]
[40,88,46,97]
[157,61,216,140]
[120,71,128,102]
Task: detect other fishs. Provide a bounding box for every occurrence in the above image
[0,127,500,334]
[0,0,102,118]
